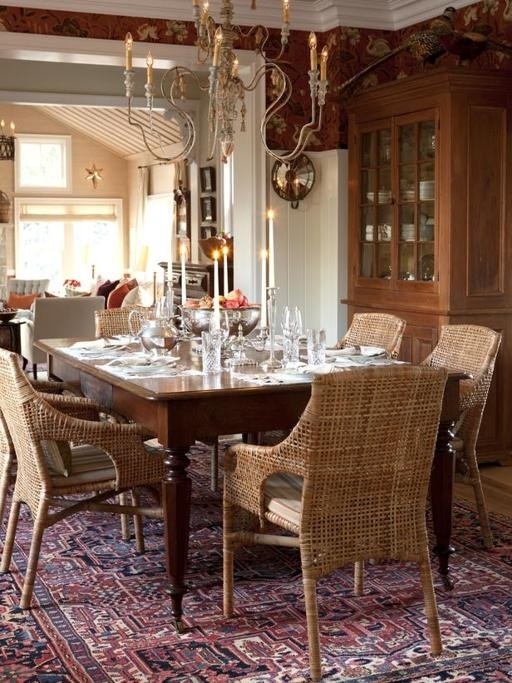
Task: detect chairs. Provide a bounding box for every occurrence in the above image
[223,367,442,682]
[4,277,106,381]
[0,305,500,612]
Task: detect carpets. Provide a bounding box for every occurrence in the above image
[0,439,511,682]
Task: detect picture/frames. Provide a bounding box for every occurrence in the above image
[198,166,216,238]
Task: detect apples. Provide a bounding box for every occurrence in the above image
[185,288,248,309]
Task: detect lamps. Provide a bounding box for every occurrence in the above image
[121,0,330,164]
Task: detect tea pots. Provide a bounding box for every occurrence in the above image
[127,309,180,355]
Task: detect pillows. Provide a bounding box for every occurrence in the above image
[90,274,144,308]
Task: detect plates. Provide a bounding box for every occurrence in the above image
[399,222,434,243]
[120,355,180,372]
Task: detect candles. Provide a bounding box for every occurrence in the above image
[212,244,230,334]
[260,208,275,327]
[167,242,187,308]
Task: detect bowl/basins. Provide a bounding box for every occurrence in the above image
[177,304,261,352]
[365,191,392,206]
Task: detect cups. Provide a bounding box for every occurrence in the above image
[160,296,174,318]
[306,328,327,368]
[201,331,221,376]
[209,310,229,345]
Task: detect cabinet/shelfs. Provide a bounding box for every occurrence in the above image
[158,261,233,330]
[346,66,511,468]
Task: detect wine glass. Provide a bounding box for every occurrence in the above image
[281,305,303,360]
